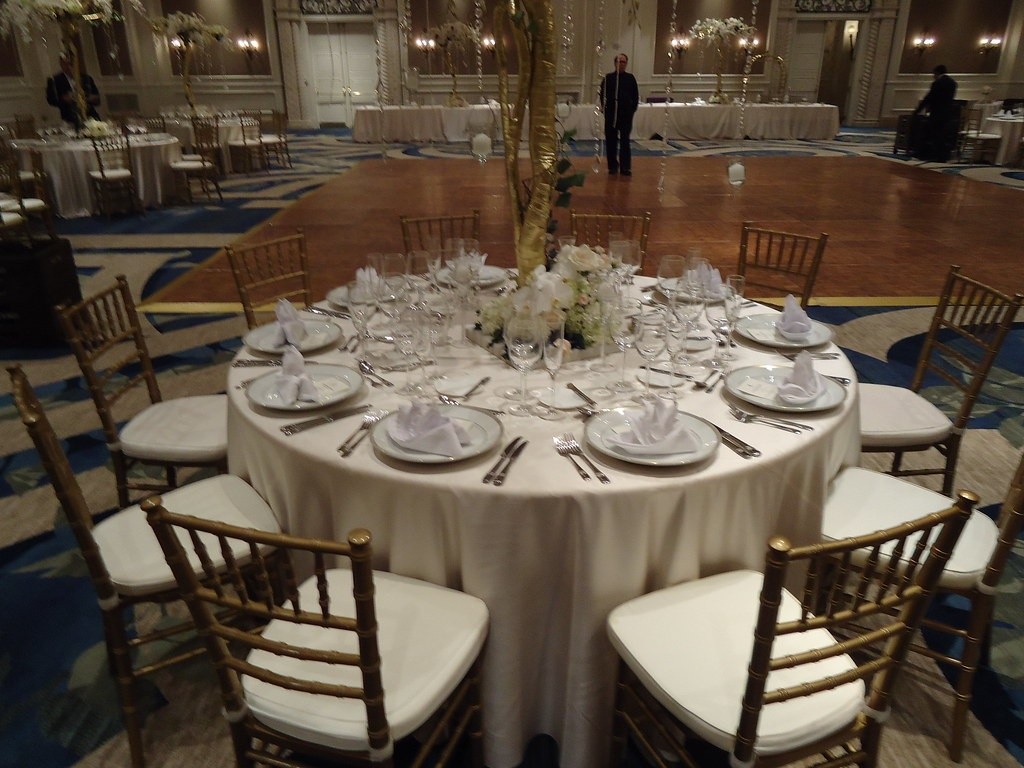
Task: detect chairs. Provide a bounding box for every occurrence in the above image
[958,107,1001,164]
[13,207,1024,768]
[0,107,297,253]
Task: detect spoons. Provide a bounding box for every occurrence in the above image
[360,361,396,389]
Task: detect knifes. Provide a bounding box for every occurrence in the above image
[483,436,529,486]
[233,358,320,367]
[705,418,762,460]
[281,404,372,437]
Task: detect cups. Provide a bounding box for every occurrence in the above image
[557,235,575,248]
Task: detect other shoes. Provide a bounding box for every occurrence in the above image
[620,169,632,175]
[608,168,619,176]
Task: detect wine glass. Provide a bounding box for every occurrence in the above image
[588,232,746,404]
[347,238,482,398]
[503,304,570,421]
[34,122,152,146]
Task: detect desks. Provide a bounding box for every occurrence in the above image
[352,103,839,143]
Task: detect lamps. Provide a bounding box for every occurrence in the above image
[975,28,1003,56]
[170,32,261,63]
[912,24,935,55]
[739,34,761,60]
[669,32,693,63]
[409,33,501,62]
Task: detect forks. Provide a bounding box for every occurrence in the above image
[338,415,376,458]
[553,432,610,485]
[728,402,814,434]
[773,347,840,361]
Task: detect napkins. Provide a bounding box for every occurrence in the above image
[386,397,475,456]
[748,294,813,344]
[267,296,308,346]
[774,349,829,404]
[602,394,704,454]
[267,348,317,404]
[686,262,724,297]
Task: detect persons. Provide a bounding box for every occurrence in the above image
[915,64,956,163]
[46,51,101,128]
[600,53,640,176]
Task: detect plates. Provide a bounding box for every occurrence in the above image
[244,364,364,410]
[654,280,735,304]
[433,330,713,409]
[242,319,343,354]
[733,313,834,348]
[725,364,848,412]
[584,407,722,467]
[325,282,395,307]
[625,272,666,287]
[370,404,504,463]
[436,264,508,286]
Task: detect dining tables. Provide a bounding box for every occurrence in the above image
[226,269,861,768]
[970,100,1024,165]
[145,115,260,174]
[7,134,182,219]
